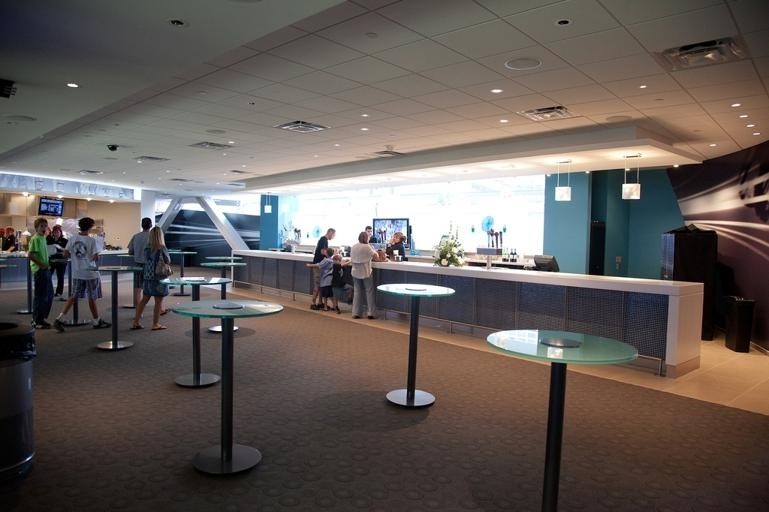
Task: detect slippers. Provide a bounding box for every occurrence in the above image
[152,324,168,330]
[130,322,143,329]
[160,306,172,316]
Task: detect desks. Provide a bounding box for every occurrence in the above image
[375,281,457,411]
[484,327,642,512]
[171,299,286,476]
[2,246,248,391]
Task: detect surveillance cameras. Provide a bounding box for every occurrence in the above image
[108,145,117,151]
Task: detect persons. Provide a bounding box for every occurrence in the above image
[306,226,406,319]
[27,218,54,329]
[129,227,171,329]
[127,217,170,316]
[54,217,113,332]
[0,224,68,296]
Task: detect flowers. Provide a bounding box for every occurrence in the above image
[431,229,467,271]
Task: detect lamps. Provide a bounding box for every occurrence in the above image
[553,158,576,203]
[619,153,643,202]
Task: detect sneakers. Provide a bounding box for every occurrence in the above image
[93,318,112,328]
[334,305,341,314]
[31,318,50,329]
[53,319,64,331]
[352,314,377,319]
[309,303,330,310]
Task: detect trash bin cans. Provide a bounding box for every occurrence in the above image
[724,294,756,353]
[0,323,39,486]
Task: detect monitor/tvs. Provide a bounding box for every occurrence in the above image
[38,198,64,216]
[373,218,412,249]
[534,255,559,272]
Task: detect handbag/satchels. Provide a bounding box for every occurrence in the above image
[155,247,173,281]
